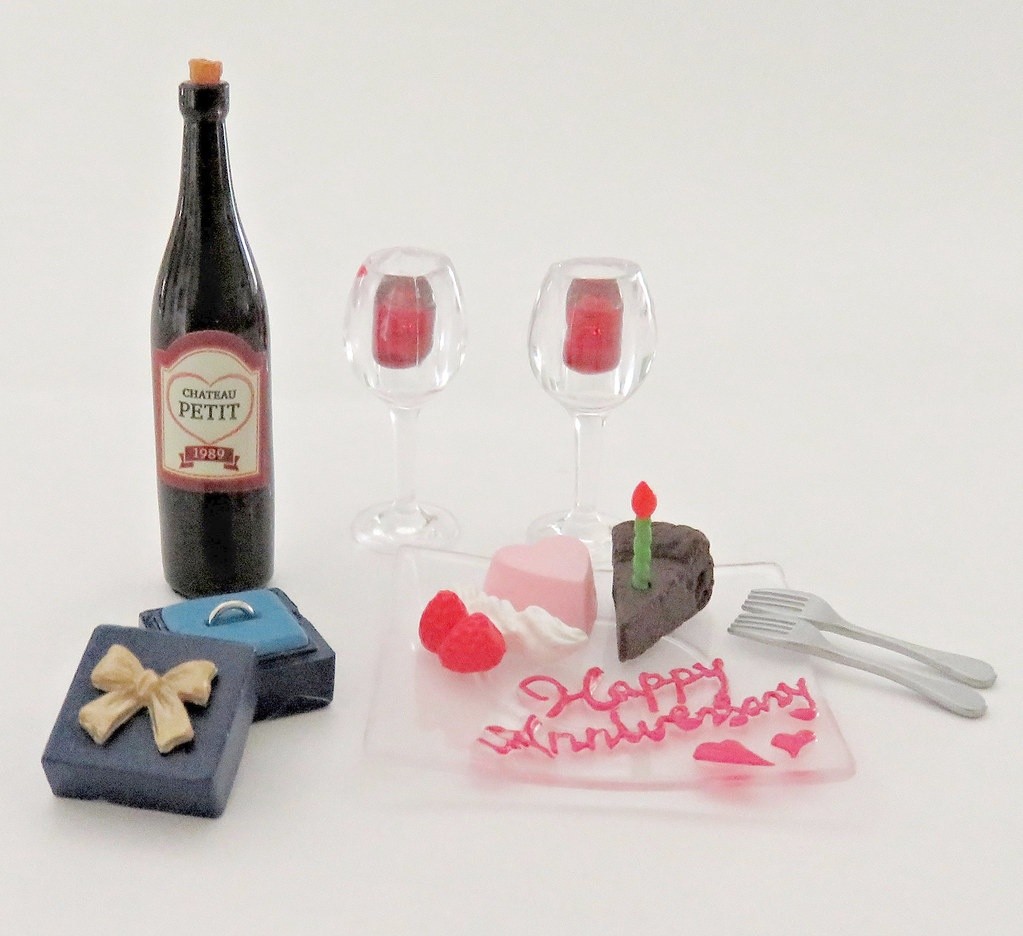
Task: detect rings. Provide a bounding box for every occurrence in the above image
[209,600,255,624]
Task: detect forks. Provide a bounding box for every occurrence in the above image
[742,586,995,688]
[726,612,988,719]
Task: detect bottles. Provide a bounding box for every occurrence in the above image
[147,59,273,602]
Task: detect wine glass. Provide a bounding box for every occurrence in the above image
[528,256,646,574]
[342,245,466,551]
